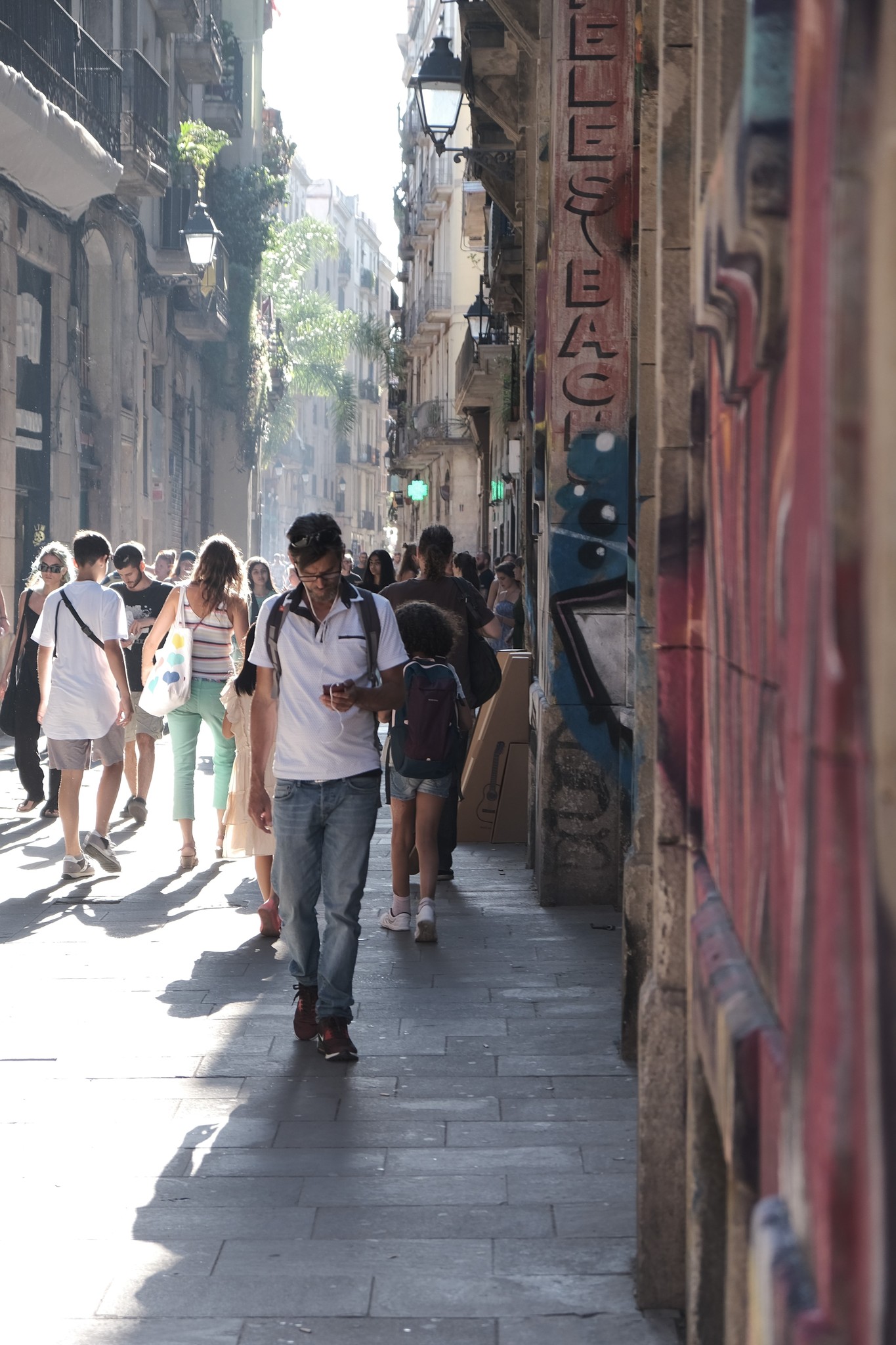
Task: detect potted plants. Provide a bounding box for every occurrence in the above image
[174,116,233,188]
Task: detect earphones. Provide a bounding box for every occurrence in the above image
[293,561,298,569]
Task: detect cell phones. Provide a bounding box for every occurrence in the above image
[323,682,344,697]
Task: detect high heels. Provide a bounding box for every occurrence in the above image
[215,835,224,859]
[178,843,199,868]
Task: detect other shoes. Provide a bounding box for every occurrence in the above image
[120,795,148,823]
[437,869,454,881]
[409,844,420,875]
[257,899,283,937]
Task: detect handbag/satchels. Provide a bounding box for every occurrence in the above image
[138,627,193,718]
[0,681,17,737]
[452,574,503,709]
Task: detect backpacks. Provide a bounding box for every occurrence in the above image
[385,654,465,806]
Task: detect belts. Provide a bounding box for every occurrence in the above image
[302,768,384,786]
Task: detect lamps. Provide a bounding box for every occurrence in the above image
[383,448,394,472]
[141,201,225,298]
[405,32,516,184]
[463,274,521,346]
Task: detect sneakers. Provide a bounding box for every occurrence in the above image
[291,981,360,1063]
[414,904,438,942]
[61,830,121,879]
[380,908,411,931]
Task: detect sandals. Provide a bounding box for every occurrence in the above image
[44,807,60,818]
[16,795,45,813]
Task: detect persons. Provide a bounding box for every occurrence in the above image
[375,523,503,884]
[30,530,134,880]
[219,622,280,937]
[0,540,524,823]
[247,512,410,1063]
[379,599,475,942]
[141,534,250,868]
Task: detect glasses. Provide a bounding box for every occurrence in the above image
[457,551,470,568]
[343,560,351,564]
[290,527,339,549]
[158,550,175,561]
[293,559,342,582]
[111,555,131,563]
[38,564,64,573]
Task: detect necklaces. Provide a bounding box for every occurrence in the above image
[504,587,517,602]
